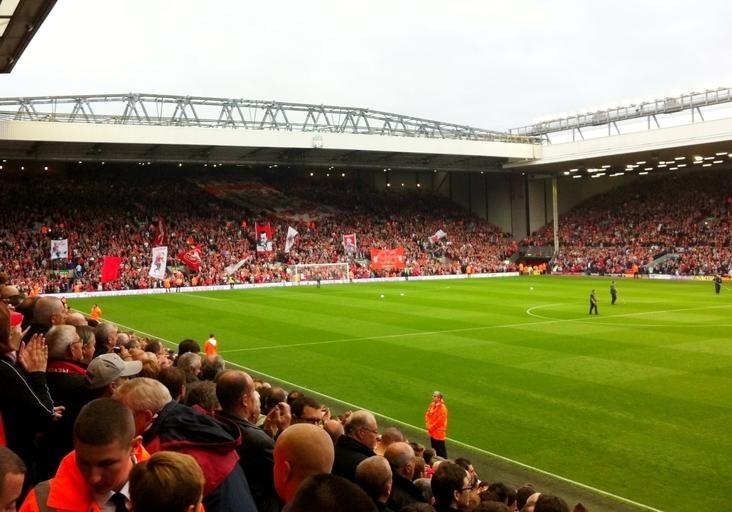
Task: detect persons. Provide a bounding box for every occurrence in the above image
[519,168,732,277]
[713,274,722,295]
[1,281,580,512]
[610,281,617,304]
[1,178,518,292]
[589,289,598,315]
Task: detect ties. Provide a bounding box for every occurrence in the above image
[108,492,131,512]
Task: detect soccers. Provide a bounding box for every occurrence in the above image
[381,295,384,298]
[401,293,404,296]
[530,287,534,290]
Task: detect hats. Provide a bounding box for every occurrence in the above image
[85,352,143,391]
[1,284,21,299]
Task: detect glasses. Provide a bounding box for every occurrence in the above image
[297,417,324,425]
